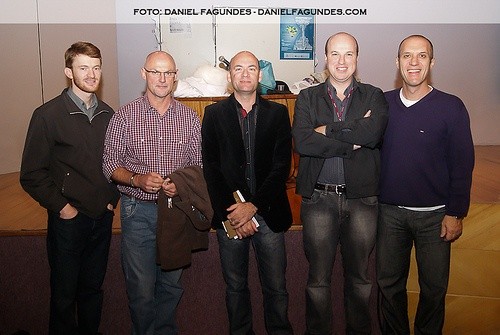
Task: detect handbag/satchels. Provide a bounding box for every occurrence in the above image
[258,60,276,95]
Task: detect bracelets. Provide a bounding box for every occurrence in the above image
[130,173,139,188]
[447,215,464,219]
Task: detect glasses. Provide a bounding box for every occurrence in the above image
[144,67,178,78]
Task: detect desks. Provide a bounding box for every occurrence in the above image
[175,94,300,179]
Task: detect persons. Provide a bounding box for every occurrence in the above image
[101,50,202,335]
[290,31,389,335]
[20,41,120,335]
[374,35,474,335]
[202,52,294,335]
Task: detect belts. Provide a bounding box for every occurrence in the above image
[314,182,349,195]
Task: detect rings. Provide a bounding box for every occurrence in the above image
[152,187,154,191]
